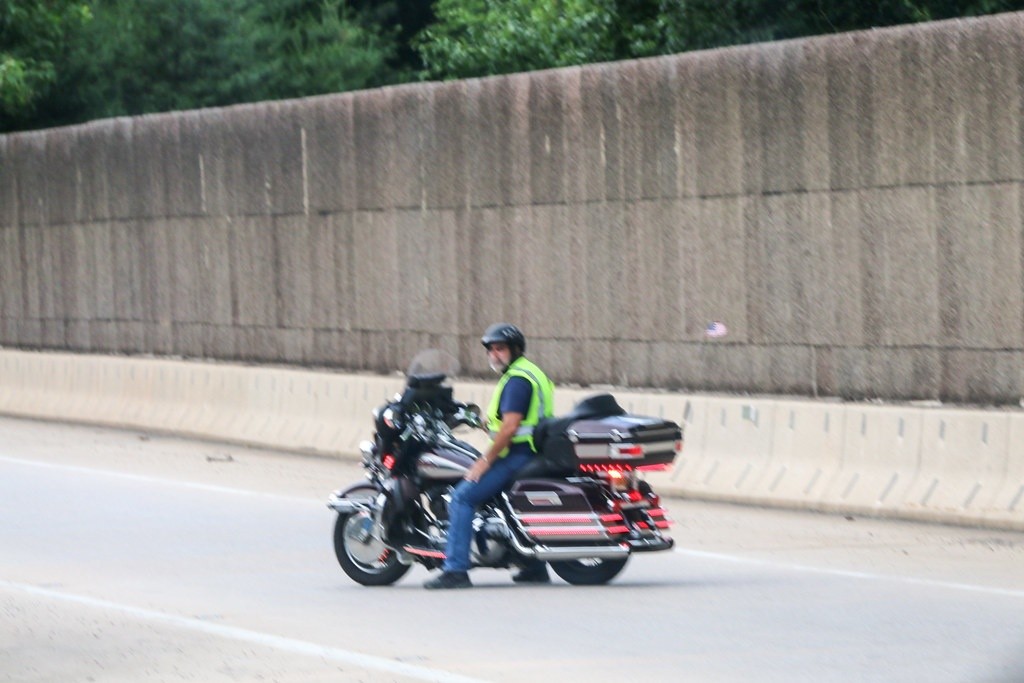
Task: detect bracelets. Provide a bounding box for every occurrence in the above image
[482,455,489,462]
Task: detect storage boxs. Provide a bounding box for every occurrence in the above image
[567,414,683,467]
[605,479,669,529]
[506,477,628,544]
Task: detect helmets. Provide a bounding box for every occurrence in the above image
[480,322,525,352]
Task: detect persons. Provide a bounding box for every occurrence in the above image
[422,322,555,591]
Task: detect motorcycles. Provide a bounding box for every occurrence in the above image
[326,347,686,586]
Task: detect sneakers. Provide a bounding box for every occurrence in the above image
[513,569,550,582]
[424,571,473,590]
